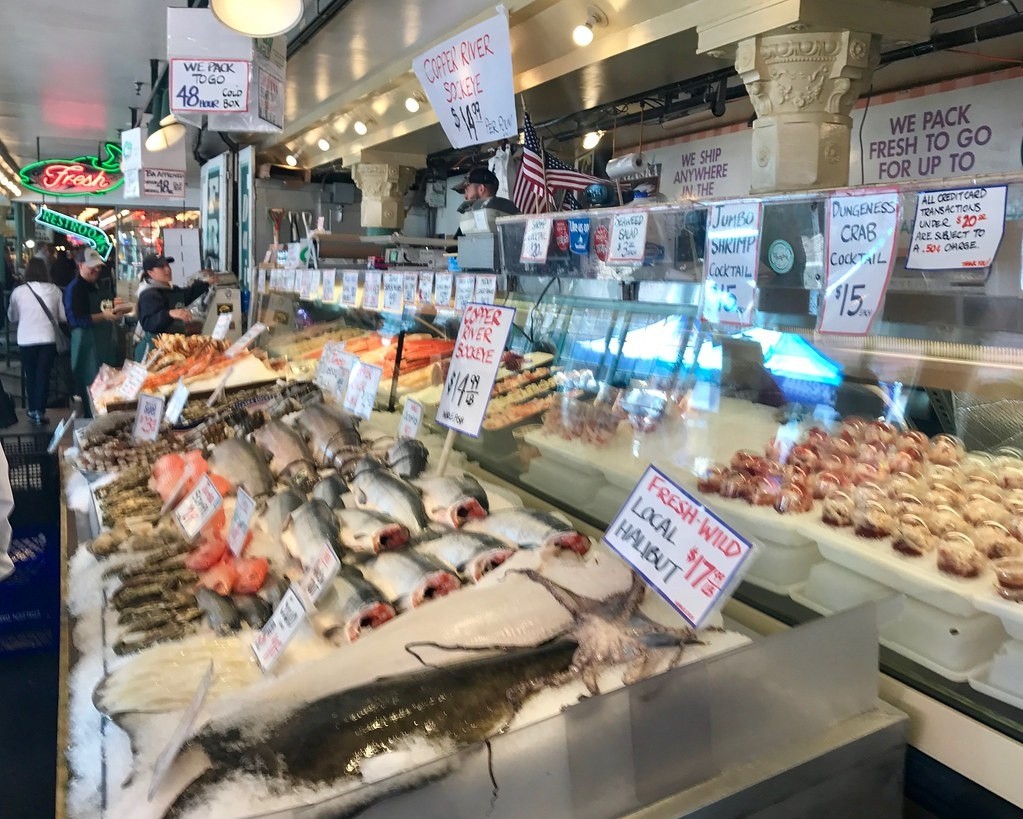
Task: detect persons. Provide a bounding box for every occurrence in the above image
[445,168,521,254]
[0,440,16,585]
[7,241,126,426]
[134,254,219,364]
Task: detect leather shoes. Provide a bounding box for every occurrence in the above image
[35,411,50,425]
[25,409,35,419]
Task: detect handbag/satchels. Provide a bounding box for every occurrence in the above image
[55,328,70,357]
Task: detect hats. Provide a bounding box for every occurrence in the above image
[450,167,499,194]
[75,247,106,268]
[143,254,174,272]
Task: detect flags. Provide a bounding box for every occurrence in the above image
[511,110,556,214]
[560,190,583,211]
[541,141,615,191]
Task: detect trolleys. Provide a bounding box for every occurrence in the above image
[0,431,61,661]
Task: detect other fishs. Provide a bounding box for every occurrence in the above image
[105,331,260,389]
[72,374,624,788]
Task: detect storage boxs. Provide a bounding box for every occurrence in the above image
[0,430,60,499]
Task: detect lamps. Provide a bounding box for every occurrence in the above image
[209,0,303,38]
[144,88,187,151]
